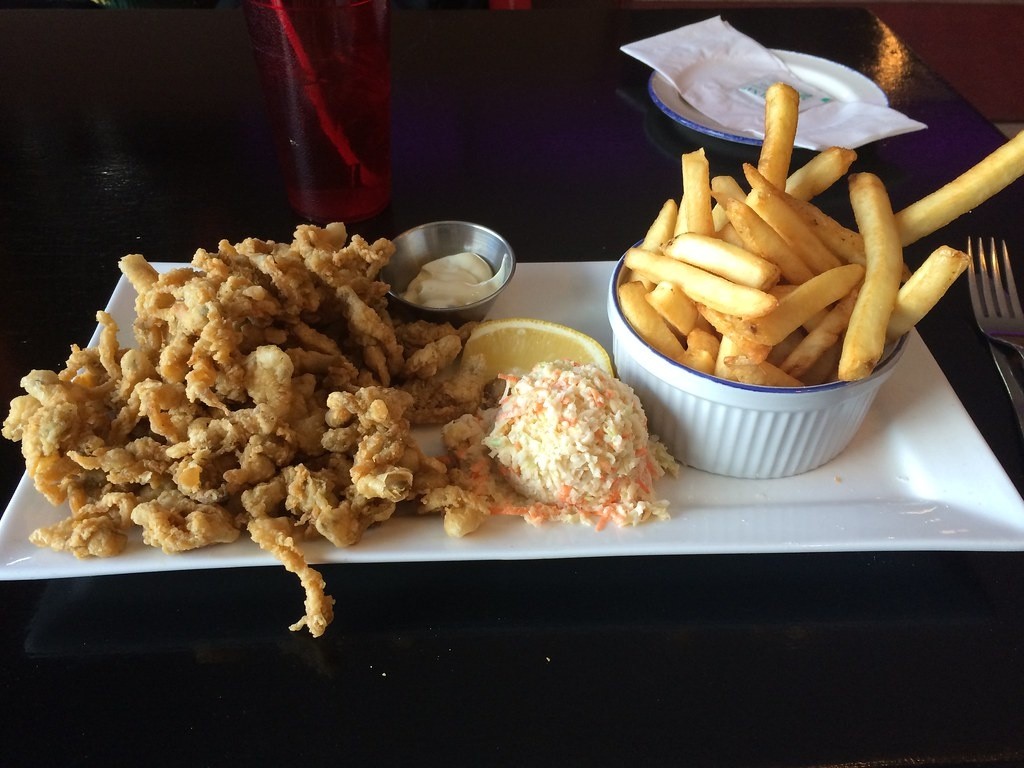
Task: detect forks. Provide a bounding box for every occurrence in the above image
[967,236,1024,365]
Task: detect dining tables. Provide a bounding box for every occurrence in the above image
[0,0,1024,768]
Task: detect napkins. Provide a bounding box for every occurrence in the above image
[619,16,928,155]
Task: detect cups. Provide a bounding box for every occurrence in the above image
[243,0,395,232]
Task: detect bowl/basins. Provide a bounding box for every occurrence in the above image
[378,221,516,326]
[605,232,909,480]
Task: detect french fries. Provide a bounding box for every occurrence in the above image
[619,83,1024,384]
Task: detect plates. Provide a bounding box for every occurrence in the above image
[646,48,889,148]
[1,261,1024,584]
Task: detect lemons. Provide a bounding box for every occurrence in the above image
[461,319,614,380]
